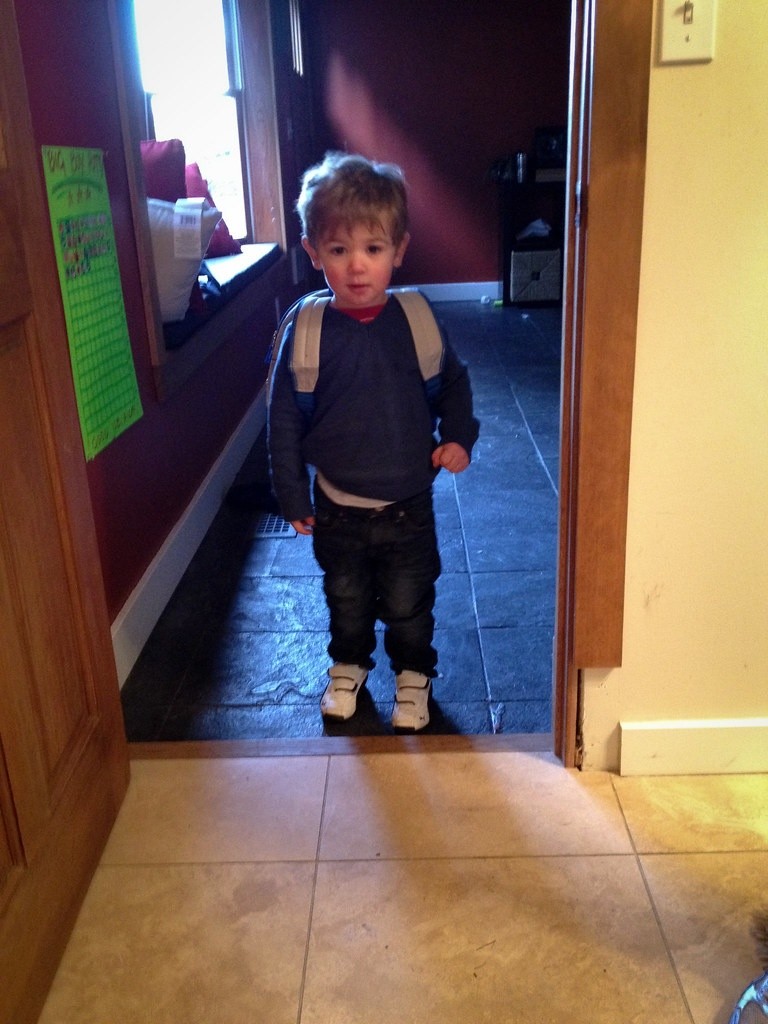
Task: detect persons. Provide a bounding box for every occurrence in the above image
[266,151,480,731]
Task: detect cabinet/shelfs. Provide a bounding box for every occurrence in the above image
[487,150,567,310]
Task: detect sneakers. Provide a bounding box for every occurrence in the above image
[391,669,430,730]
[320,662,370,722]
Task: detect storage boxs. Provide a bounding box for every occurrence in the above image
[510,249,561,301]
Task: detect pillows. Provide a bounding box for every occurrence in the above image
[148,194,225,328]
[185,163,242,259]
[139,139,186,201]
